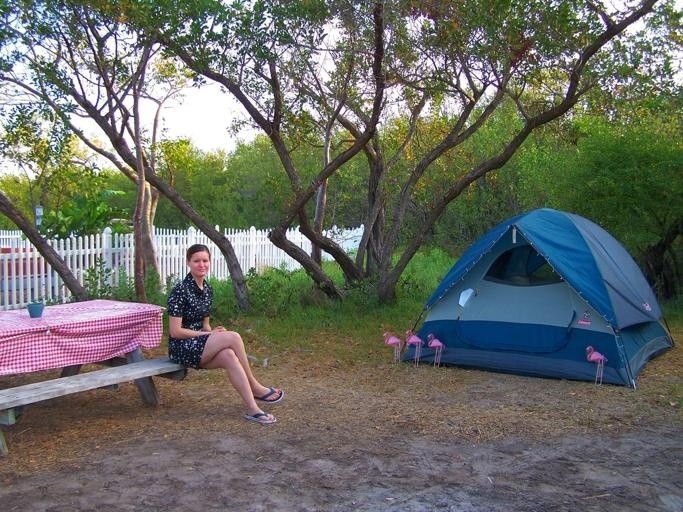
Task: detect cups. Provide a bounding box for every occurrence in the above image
[27,303,45,319]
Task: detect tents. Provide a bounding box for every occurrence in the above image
[400,209,674,388]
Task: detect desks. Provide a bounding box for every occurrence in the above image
[0,300,167,408]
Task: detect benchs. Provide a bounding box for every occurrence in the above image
[0,357,188,457]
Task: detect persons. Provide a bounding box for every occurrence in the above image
[166,245,282,423]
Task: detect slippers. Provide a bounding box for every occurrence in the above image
[254,387,284,403]
[243,412,277,424]
